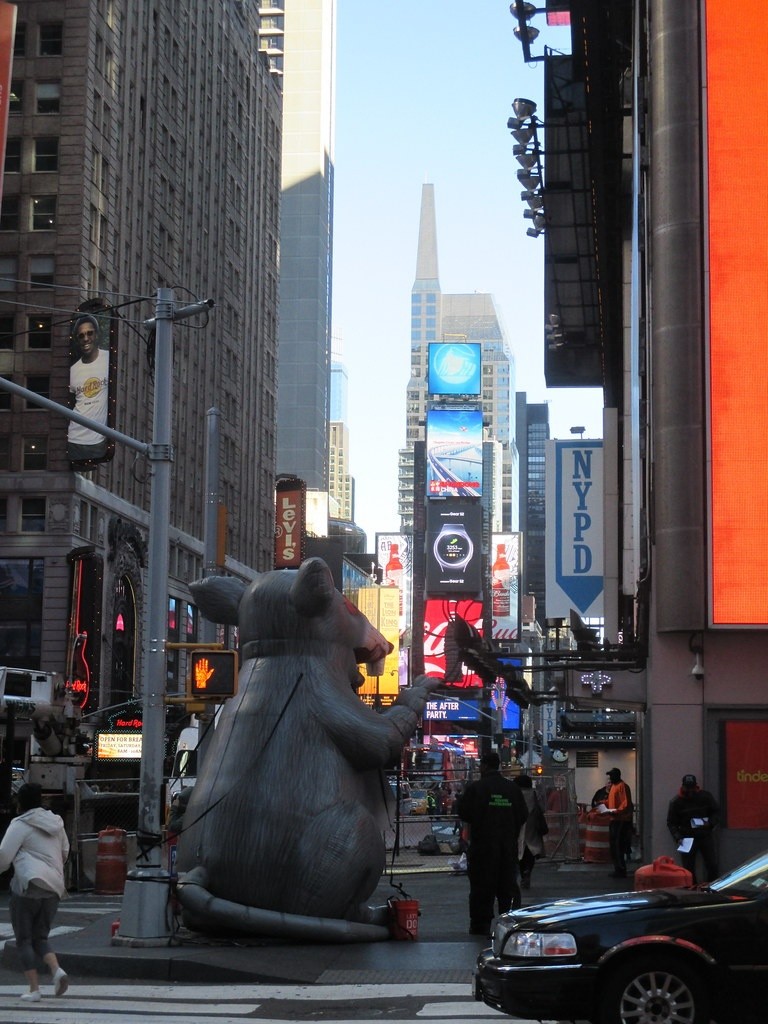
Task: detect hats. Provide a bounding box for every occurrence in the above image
[514,775,532,788]
[480,752,499,770]
[18,781,42,807]
[605,767,621,775]
[681,774,697,784]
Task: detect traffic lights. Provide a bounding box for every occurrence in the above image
[187,647,239,700]
[536,764,544,775]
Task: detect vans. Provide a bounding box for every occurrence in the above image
[388,780,412,816]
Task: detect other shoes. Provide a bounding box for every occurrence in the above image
[520,878,530,889]
[20,989,42,1002]
[54,967,70,996]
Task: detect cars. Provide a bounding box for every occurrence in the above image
[471,848,767,1024]
[410,788,435,815]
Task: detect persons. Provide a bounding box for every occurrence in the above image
[453,751,530,936]
[0,781,69,1002]
[67,314,110,460]
[591,771,613,810]
[599,767,635,880]
[400,749,576,839]
[508,774,550,889]
[665,775,724,885]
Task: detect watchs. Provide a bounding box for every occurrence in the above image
[431,522,475,584]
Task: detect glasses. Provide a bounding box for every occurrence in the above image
[76,330,95,339]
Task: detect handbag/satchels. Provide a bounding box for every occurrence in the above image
[532,791,549,835]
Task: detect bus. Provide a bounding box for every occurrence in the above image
[400,742,471,816]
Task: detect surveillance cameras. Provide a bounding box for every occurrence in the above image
[691,665,705,682]
